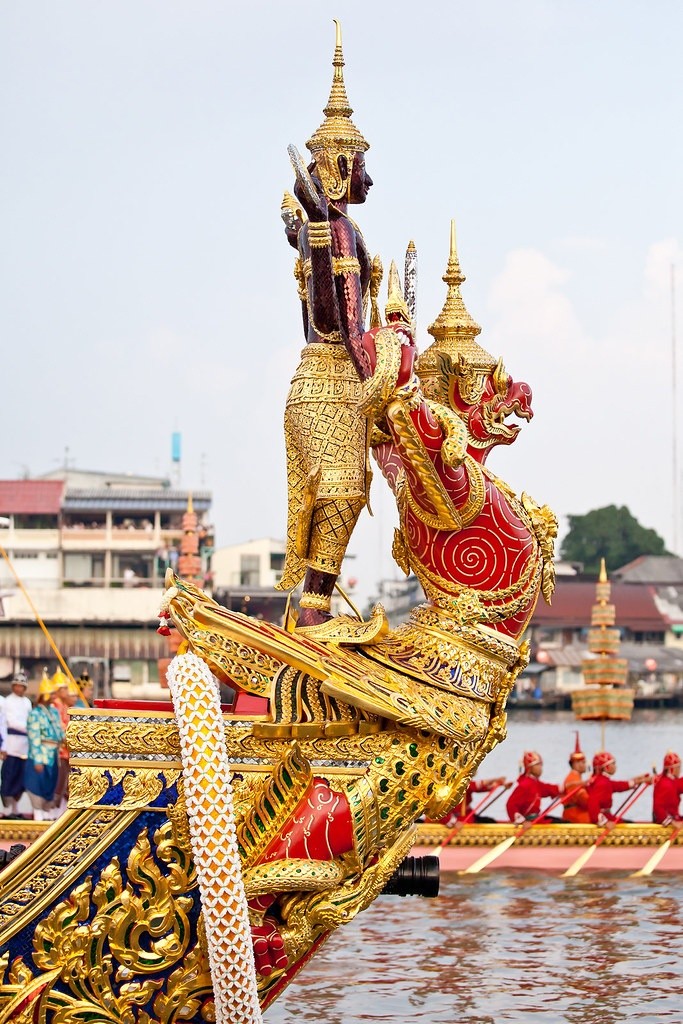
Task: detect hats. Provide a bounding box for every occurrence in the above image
[523,752,542,768]
[593,752,616,770]
[35,667,56,705]
[51,666,70,688]
[664,749,681,769]
[569,731,585,763]
[11,668,29,688]
[68,678,79,696]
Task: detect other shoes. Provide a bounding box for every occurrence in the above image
[0,813,30,820]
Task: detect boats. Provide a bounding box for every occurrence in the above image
[403,816,683,870]
[0,18,562,1024]
[501,632,682,710]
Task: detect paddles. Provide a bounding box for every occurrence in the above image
[425,781,515,858]
[625,819,683,878]
[465,781,589,874]
[559,782,651,878]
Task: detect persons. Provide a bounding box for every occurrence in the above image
[0,664,32,820]
[562,731,593,824]
[76,670,94,708]
[653,748,683,829]
[585,751,652,830]
[424,776,506,832]
[24,665,79,820]
[506,752,572,829]
[276,17,389,649]
